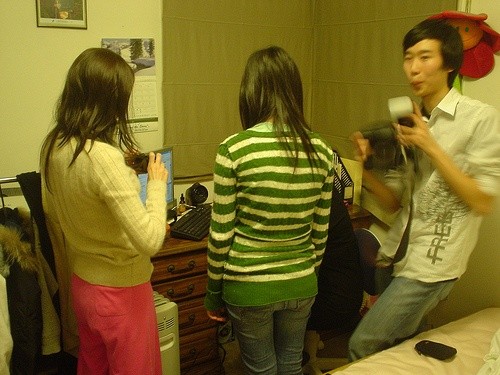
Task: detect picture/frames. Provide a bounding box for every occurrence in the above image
[36,0,88,30]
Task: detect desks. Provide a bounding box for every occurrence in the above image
[348,202,371,230]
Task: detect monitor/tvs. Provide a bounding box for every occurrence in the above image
[137,145,177,211]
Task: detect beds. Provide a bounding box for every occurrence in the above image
[321,307,500,375]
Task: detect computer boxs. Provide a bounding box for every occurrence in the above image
[153,290,181,375]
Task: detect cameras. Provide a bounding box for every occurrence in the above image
[124,154,156,175]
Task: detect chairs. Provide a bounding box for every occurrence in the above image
[301,227,382,375]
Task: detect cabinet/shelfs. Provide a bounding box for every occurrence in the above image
[151,229,225,375]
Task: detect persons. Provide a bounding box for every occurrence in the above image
[347,18,500,362]
[39,48,169,375]
[206,47,334,375]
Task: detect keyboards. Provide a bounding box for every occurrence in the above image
[171,203,212,241]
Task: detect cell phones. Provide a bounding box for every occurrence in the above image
[416,340,457,360]
